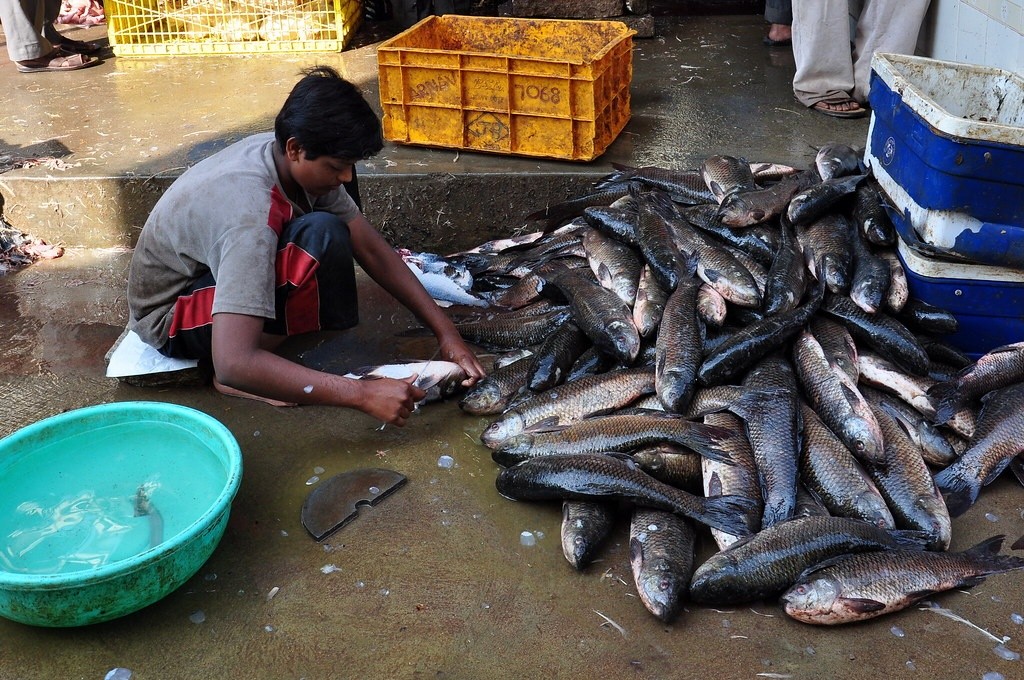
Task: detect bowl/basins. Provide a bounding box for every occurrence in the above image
[0,399,244,629]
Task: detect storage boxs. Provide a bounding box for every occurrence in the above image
[104,0,359,57]
[864,51,1024,271]
[377,14,637,161]
[896,228,1024,363]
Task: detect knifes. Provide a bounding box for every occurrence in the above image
[380,341,447,432]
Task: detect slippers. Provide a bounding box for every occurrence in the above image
[60,37,101,55]
[793,96,867,117]
[15,49,98,72]
[763,34,793,46]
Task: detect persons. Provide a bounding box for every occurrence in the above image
[0,0,101,74]
[764,0,931,118]
[127,66,486,427]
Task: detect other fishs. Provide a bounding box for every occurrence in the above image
[394,138,1023,627]
[345,358,467,406]
